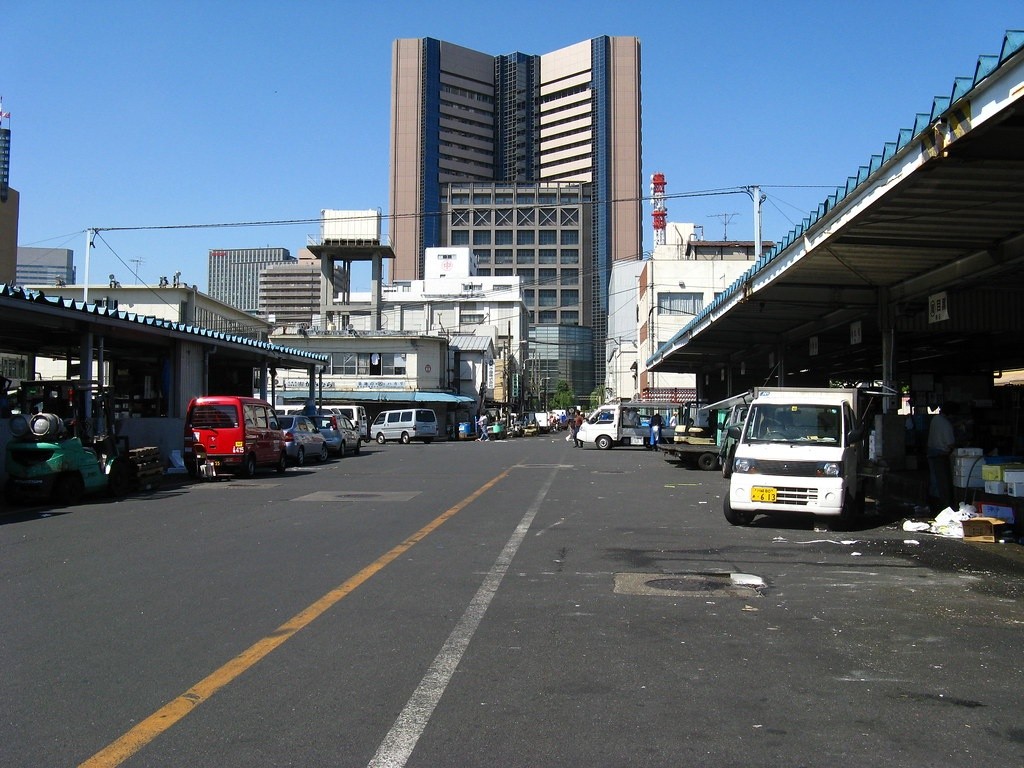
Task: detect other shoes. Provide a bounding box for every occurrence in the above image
[485,439,490,442]
[478,439,482,442]
[573,445,578,448]
[579,446,584,448]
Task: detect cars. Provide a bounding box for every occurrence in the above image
[275,414,328,467]
[305,414,361,458]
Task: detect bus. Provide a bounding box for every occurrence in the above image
[274,405,368,441]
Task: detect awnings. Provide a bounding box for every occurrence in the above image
[264,392,477,405]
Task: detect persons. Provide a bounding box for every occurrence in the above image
[476,411,490,442]
[600,412,612,420]
[928,405,962,510]
[649,414,666,451]
[670,413,677,427]
[524,416,528,425]
[685,418,694,436]
[550,412,568,432]
[574,411,583,448]
[511,416,514,438]
[0,374,12,416]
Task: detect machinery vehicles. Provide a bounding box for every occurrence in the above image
[6,379,173,511]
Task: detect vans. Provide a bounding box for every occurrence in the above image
[184,395,287,482]
[370,408,439,444]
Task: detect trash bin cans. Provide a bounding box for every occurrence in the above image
[459,421,472,435]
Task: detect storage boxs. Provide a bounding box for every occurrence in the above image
[953,448,1024,543]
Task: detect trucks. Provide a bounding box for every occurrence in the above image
[656,404,764,478]
[698,386,897,530]
[446,405,587,442]
[575,400,684,451]
